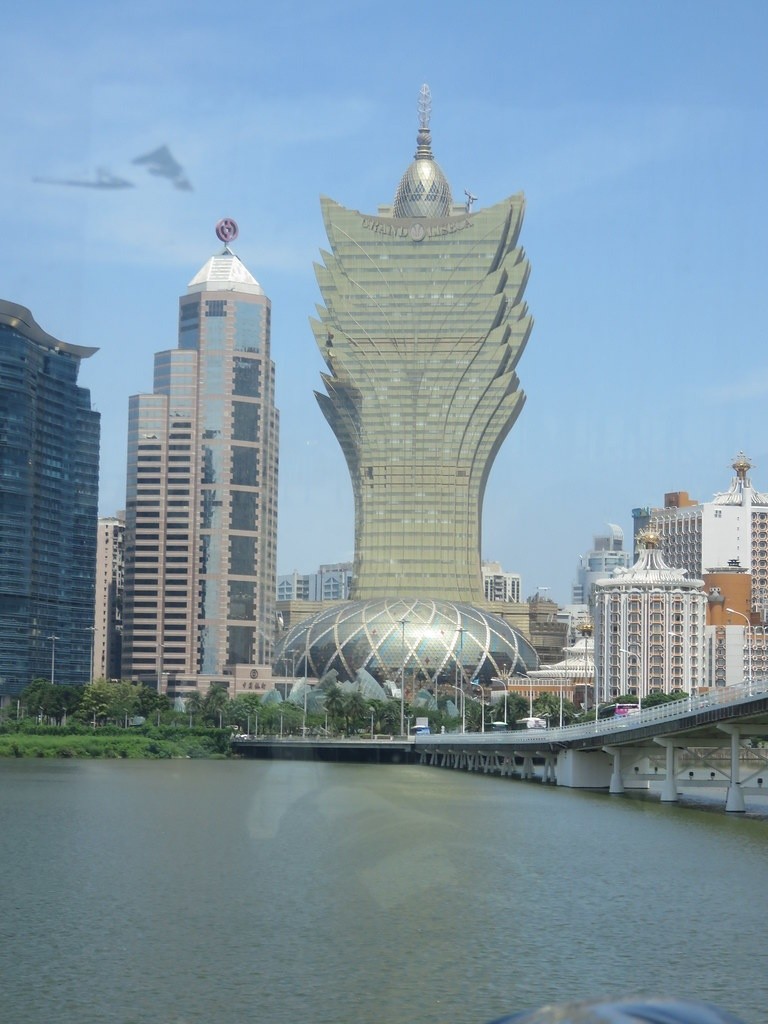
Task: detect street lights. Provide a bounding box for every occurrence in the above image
[581,658,600,732]
[281,657,295,699]
[491,677,508,724]
[396,617,412,735]
[35,703,378,736]
[455,625,469,720]
[515,672,533,718]
[618,649,643,711]
[469,680,485,732]
[544,665,563,727]
[47,631,61,686]
[726,607,755,697]
[444,683,466,734]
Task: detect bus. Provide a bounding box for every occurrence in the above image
[408,726,431,736]
[516,718,546,729]
[573,683,604,710]
[599,702,641,719]
[484,721,508,732]
